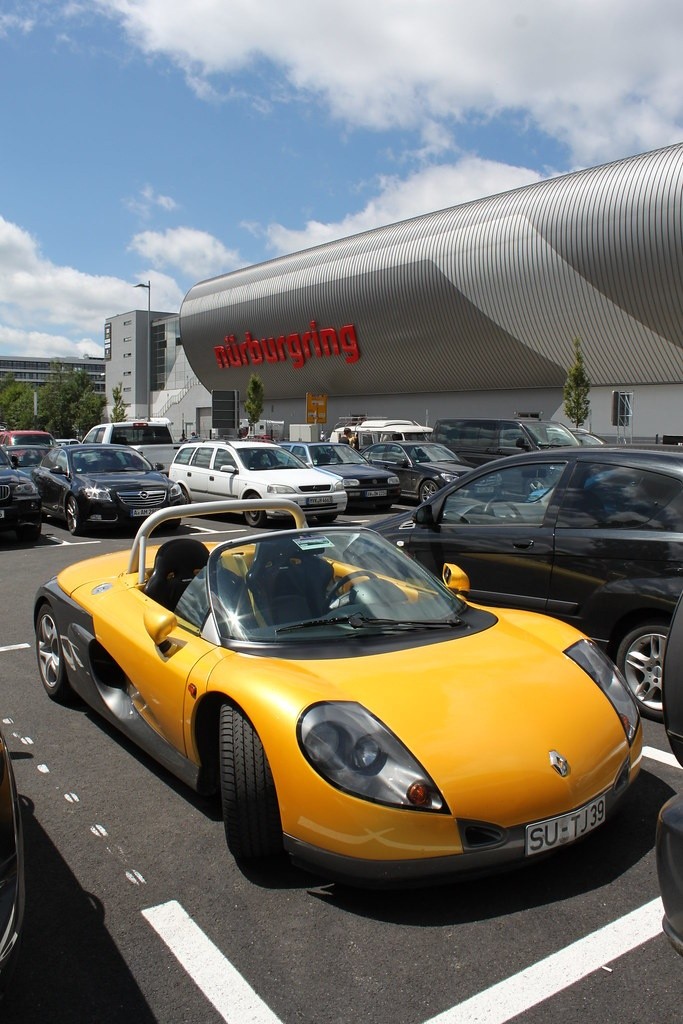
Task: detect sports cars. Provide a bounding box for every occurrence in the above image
[34,496,647,891]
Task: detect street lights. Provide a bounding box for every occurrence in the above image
[133,280,155,422]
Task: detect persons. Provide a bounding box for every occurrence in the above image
[179,434,188,442]
[352,434,359,449]
[191,432,198,438]
[339,428,352,444]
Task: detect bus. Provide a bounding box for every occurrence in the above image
[330,420,434,452]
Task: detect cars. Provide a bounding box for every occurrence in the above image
[352,442,481,503]
[562,427,607,450]
[0,430,59,466]
[341,440,683,723]
[52,438,80,453]
[277,441,402,511]
[29,443,186,536]
[0,444,44,539]
[0,727,28,966]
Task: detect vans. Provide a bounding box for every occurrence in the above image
[428,418,582,480]
[81,421,176,484]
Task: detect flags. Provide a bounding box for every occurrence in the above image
[133,284,149,288]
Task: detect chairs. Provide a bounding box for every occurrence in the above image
[143,538,245,650]
[245,530,343,633]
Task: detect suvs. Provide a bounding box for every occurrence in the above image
[168,440,348,525]
